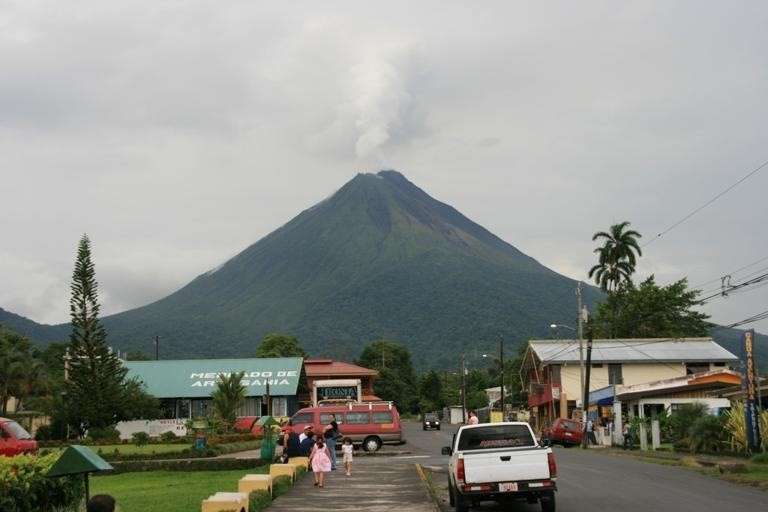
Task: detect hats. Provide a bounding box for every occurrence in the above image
[304,425,313,430]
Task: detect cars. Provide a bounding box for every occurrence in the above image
[421,410,441,432]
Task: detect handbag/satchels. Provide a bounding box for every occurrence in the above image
[332,430,343,440]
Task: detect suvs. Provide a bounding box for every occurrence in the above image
[541,415,580,449]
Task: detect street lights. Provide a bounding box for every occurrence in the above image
[151,335,160,363]
[452,370,467,424]
[543,319,586,416]
[481,353,504,414]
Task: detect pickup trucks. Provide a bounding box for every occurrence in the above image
[444,419,560,511]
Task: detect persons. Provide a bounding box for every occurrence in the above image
[282,425,300,464]
[323,414,338,470]
[468,411,479,425]
[587,420,596,445]
[622,420,633,450]
[308,437,332,488]
[341,437,356,476]
[299,432,316,456]
[299,425,313,444]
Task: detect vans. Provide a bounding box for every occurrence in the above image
[280,397,408,456]
[0,416,41,455]
